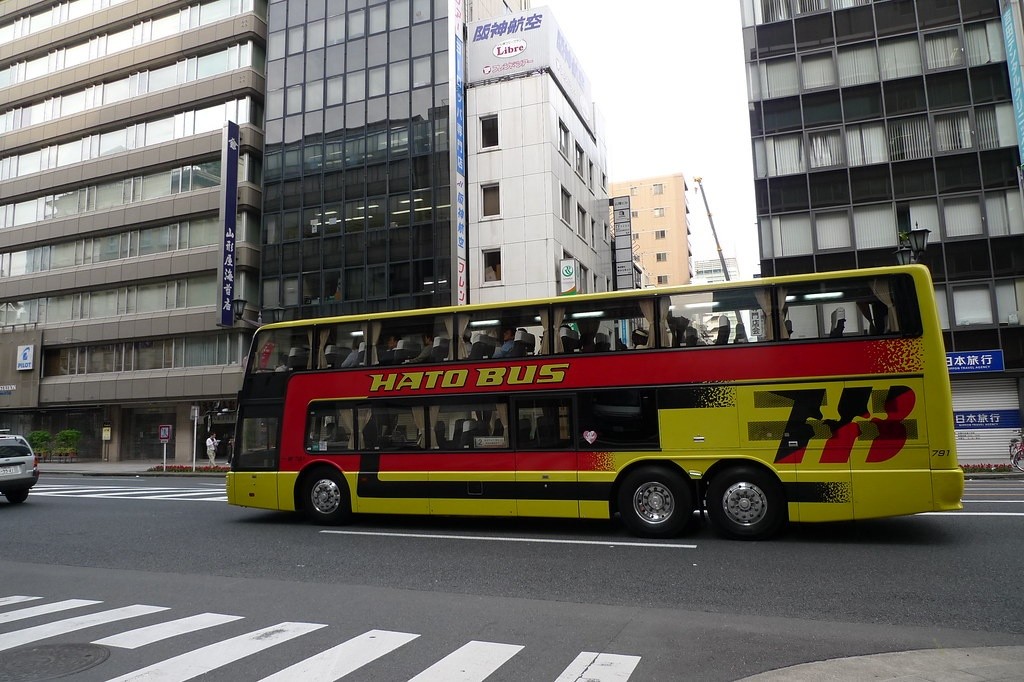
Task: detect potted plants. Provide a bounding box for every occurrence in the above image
[52,447,80,457]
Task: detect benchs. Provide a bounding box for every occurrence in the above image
[287,307,846,369]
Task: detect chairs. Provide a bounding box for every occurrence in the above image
[332,426,346,441]
[363,417,555,446]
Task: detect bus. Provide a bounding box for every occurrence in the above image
[202,266,969,540]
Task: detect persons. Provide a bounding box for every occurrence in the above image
[206,432,218,467]
[225,441,233,464]
[323,422,335,441]
[274,303,710,376]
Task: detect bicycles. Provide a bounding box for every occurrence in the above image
[1009,429,1024,472]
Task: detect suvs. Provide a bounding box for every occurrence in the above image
[0,434,40,505]
